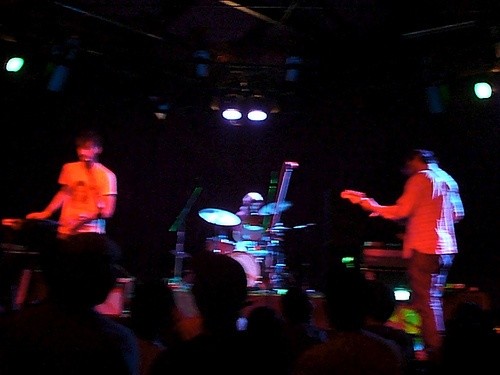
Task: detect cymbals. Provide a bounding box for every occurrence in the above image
[260,202,292,215]
[199,208,241,226]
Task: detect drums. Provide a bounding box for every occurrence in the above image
[205,238,235,254]
[226,251,261,287]
[248,250,276,276]
[242,225,264,240]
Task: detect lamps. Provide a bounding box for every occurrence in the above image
[0,52,26,74]
[247,95,269,123]
[473,79,500,101]
[220,95,244,121]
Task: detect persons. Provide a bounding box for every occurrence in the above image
[1,231,500,372]
[24,135,118,239]
[233,191,270,241]
[359,147,465,353]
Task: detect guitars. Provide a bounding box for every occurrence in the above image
[341,190,457,222]
